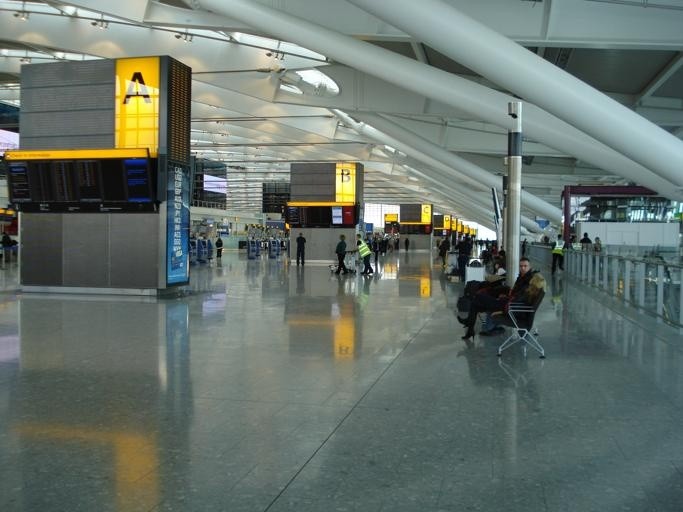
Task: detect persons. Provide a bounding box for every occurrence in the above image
[1,232,12,263]
[215,238,223,258]
[355,233,409,275]
[439,232,602,339]
[335,234,348,276]
[296,232,306,267]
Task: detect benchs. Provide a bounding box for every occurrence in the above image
[497,290,546,363]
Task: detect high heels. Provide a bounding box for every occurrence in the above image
[457,315,475,341]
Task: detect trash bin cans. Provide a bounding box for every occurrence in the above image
[465,258,485,282]
